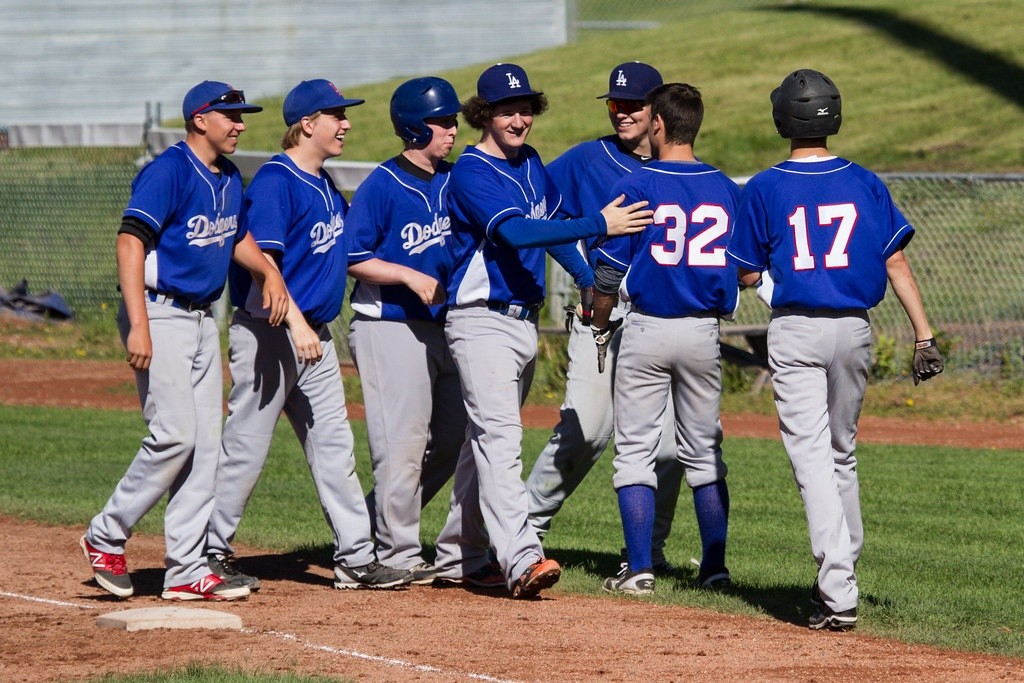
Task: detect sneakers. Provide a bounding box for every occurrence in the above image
[161,575,249,601]
[603,562,655,595]
[440,563,507,587]
[652,560,677,576]
[206,555,260,591]
[810,574,825,605]
[512,559,560,598]
[809,605,857,629]
[333,562,415,589]
[695,567,730,591]
[80,535,134,597]
[408,563,441,585]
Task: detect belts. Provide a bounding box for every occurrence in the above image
[488,300,539,319]
[149,290,209,311]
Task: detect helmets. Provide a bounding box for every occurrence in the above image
[389,77,468,146]
[770,68,843,138]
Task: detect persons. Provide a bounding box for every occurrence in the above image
[490,62,683,575]
[724,69,944,626]
[204,80,416,590]
[430,65,653,601]
[586,82,742,598]
[77,80,288,600]
[345,76,463,583]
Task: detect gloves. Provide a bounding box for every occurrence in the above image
[911,338,944,385]
[581,287,595,324]
[589,317,623,374]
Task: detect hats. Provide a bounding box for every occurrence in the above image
[183,80,263,121]
[478,64,543,102]
[282,79,364,127]
[596,61,663,99]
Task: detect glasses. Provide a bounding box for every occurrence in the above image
[605,100,642,112]
[191,91,245,115]
